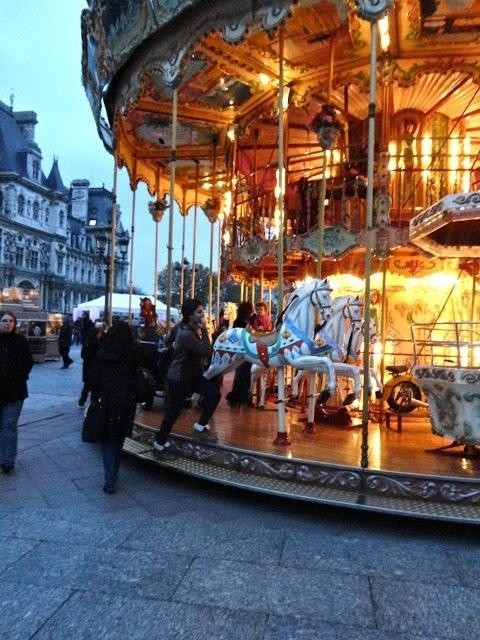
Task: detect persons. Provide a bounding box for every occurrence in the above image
[80,320,145,494]
[60,297,273,460]
[0,311,34,473]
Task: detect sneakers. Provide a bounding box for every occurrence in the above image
[193,423,219,443]
[153,441,177,460]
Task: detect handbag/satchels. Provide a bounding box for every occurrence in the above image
[135,365,158,403]
